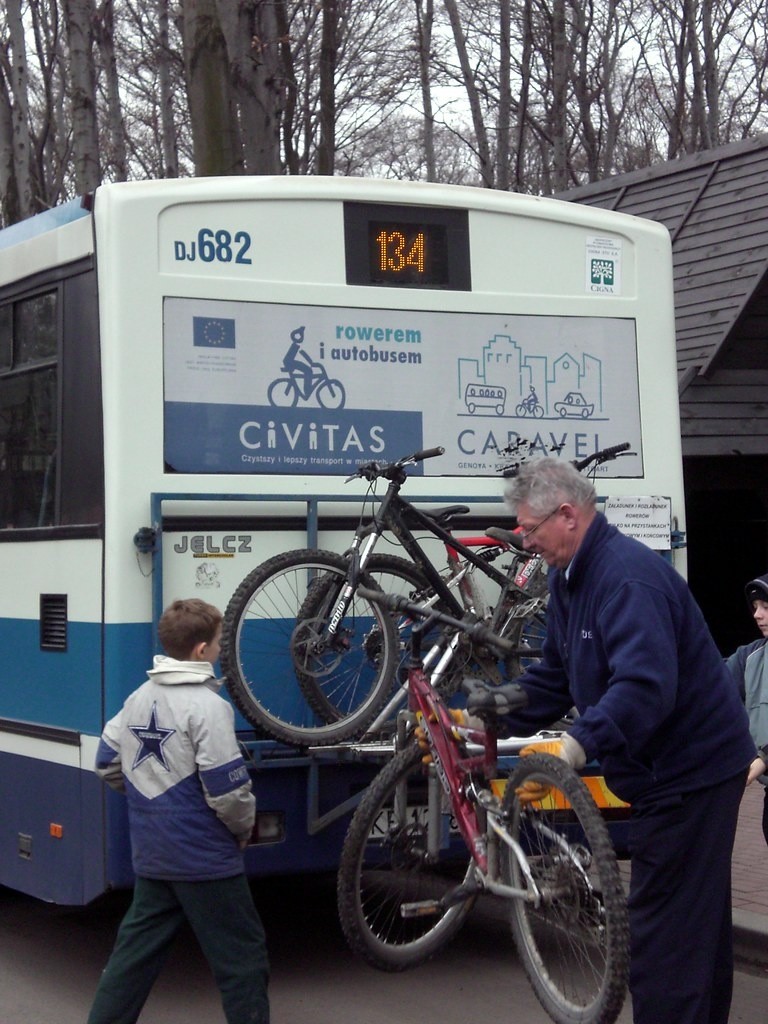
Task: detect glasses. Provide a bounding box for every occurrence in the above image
[519,503,575,540]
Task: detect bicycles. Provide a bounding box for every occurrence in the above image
[336,586,633,1024]
[218,441,640,757]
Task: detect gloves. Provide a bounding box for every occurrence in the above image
[415,707,484,764]
[514,731,586,801]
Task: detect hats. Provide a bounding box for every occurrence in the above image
[749,574,768,603]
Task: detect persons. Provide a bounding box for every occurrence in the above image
[87,598,270,1024]
[414,456,768,1024]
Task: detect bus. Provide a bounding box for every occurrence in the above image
[0,173,692,909]
